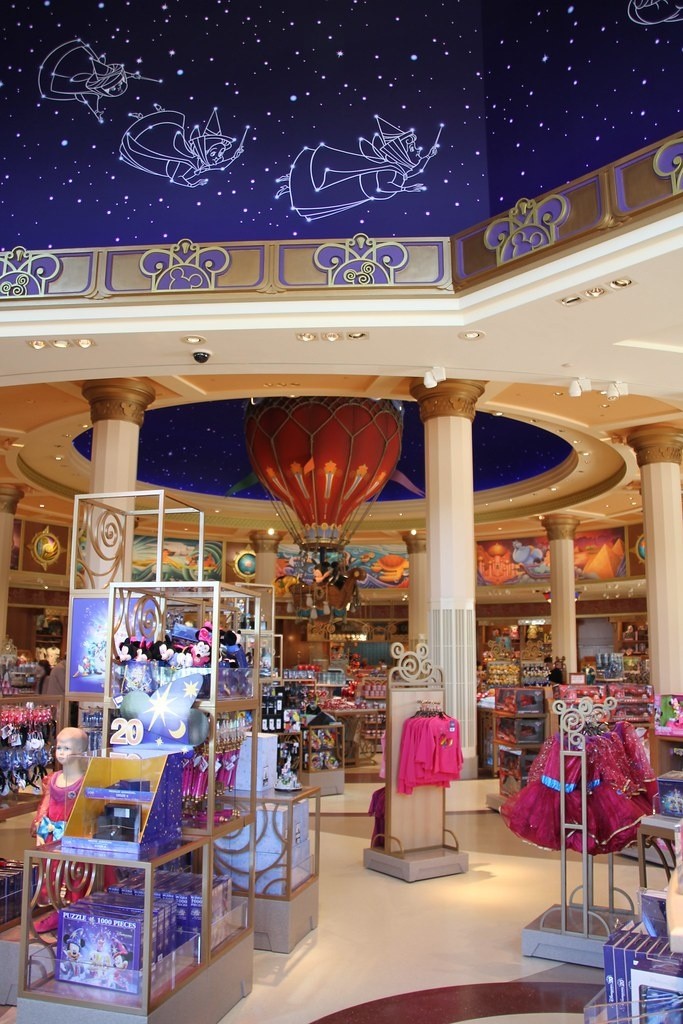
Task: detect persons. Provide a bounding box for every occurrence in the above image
[30,727,118,934]
[360,658,367,669]
[544,656,563,684]
[36,643,60,666]
[379,659,387,670]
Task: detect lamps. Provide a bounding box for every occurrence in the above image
[606,382,630,401]
[570,377,592,397]
[423,365,446,389]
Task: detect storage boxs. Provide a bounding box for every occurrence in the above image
[0,685,308,996]
[497,746,537,777]
[602,887,683,1023]
[496,688,544,714]
[495,718,545,746]
[656,771,683,818]
[553,684,654,723]
[653,694,683,737]
[499,774,525,796]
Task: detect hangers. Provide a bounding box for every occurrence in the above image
[572,705,614,736]
[413,700,446,718]
[38,644,43,649]
[50,643,57,650]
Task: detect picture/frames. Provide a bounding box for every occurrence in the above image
[569,672,587,686]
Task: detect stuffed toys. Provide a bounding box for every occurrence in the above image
[116,621,241,667]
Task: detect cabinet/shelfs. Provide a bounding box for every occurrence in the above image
[284,620,553,797]
[30,611,67,671]
[18,487,318,1024]
[637,728,683,891]
[610,616,648,658]
